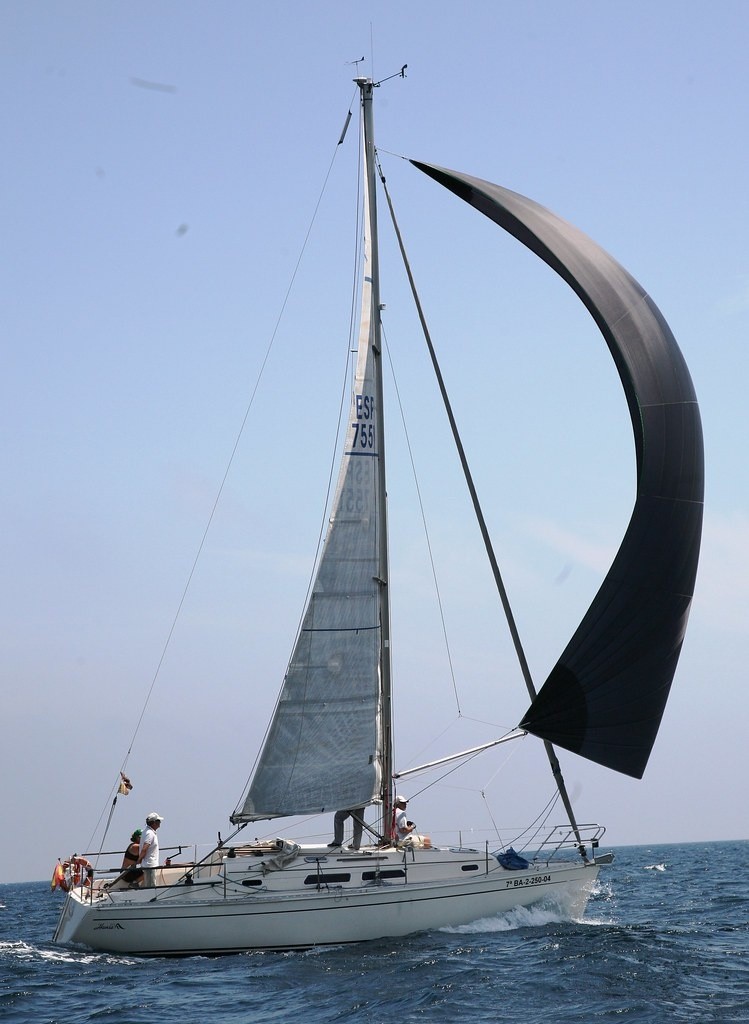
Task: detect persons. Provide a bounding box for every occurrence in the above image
[327,808,364,851]
[394,794,431,849]
[120,828,144,888]
[139,813,165,887]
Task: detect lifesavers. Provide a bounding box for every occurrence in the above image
[60,857,94,894]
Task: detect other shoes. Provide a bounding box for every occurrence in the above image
[327,842,342,847]
[348,844,359,850]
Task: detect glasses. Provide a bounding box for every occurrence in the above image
[156,820,161,823]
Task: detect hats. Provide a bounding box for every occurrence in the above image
[394,795,410,804]
[134,828,143,837]
[146,813,164,823]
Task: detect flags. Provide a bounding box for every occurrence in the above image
[117,772,133,795]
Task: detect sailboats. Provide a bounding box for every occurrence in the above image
[43,79,705,961]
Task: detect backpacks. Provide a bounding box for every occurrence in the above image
[497,847,529,870]
